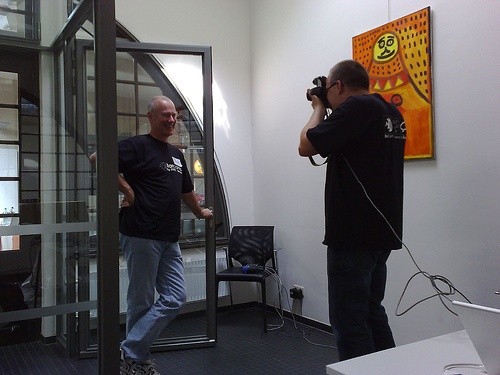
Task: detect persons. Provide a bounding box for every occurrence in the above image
[299,59,406,363]
[90,97,213,371]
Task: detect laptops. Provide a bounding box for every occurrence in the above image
[452,301,500,375]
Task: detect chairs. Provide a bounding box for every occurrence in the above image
[216,225,283,332]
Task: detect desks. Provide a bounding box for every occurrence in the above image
[326,329,498,375]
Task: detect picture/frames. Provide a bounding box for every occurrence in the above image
[351,7,434,161]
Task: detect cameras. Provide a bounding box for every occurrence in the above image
[306,76,330,108]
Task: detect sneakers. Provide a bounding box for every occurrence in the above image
[140,352,160,375]
[120,348,143,375]
